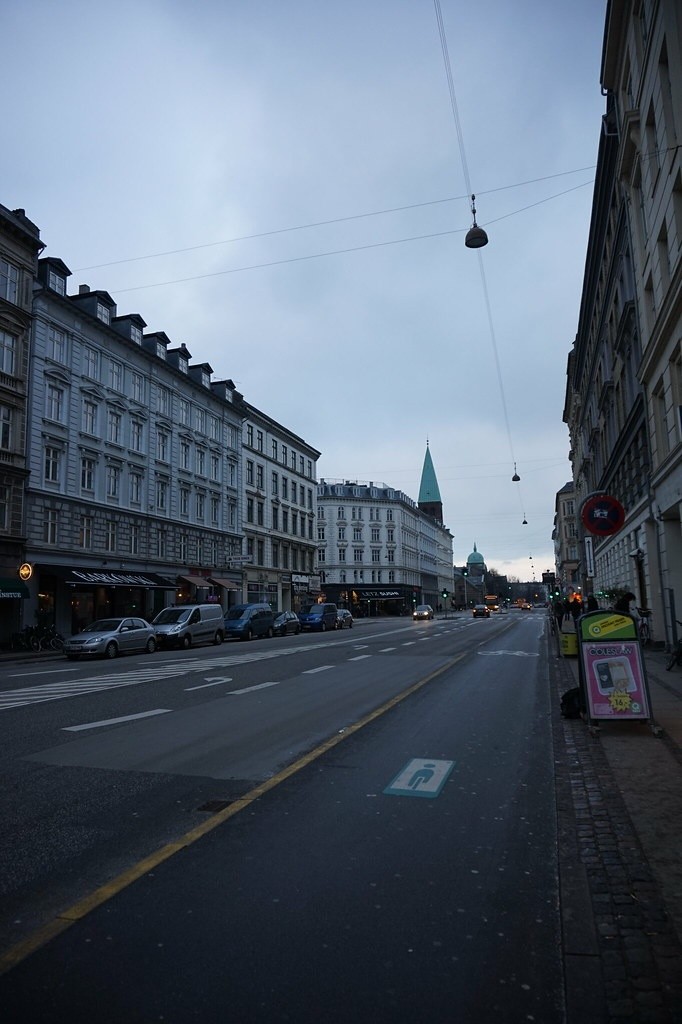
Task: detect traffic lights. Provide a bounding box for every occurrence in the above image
[441,590,449,598]
[462,568,468,577]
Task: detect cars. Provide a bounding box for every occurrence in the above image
[514,598,531,610]
[473,605,491,618]
[272,611,302,635]
[335,608,356,628]
[63,617,157,658]
[413,604,434,621]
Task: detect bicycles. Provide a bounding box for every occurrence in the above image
[14,623,66,653]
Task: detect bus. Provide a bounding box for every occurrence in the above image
[486,595,500,610]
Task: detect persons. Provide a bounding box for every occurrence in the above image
[614,592,636,612]
[554,600,564,631]
[570,599,581,629]
[563,598,571,622]
[588,592,598,613]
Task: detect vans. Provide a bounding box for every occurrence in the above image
[224,603,274,639]
[298,602,341,631]
[149,603,226,648]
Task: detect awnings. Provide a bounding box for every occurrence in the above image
[55,570,179,591]
[211,579,243,592]
[180,575,213,589]
[0,578,30,599]
